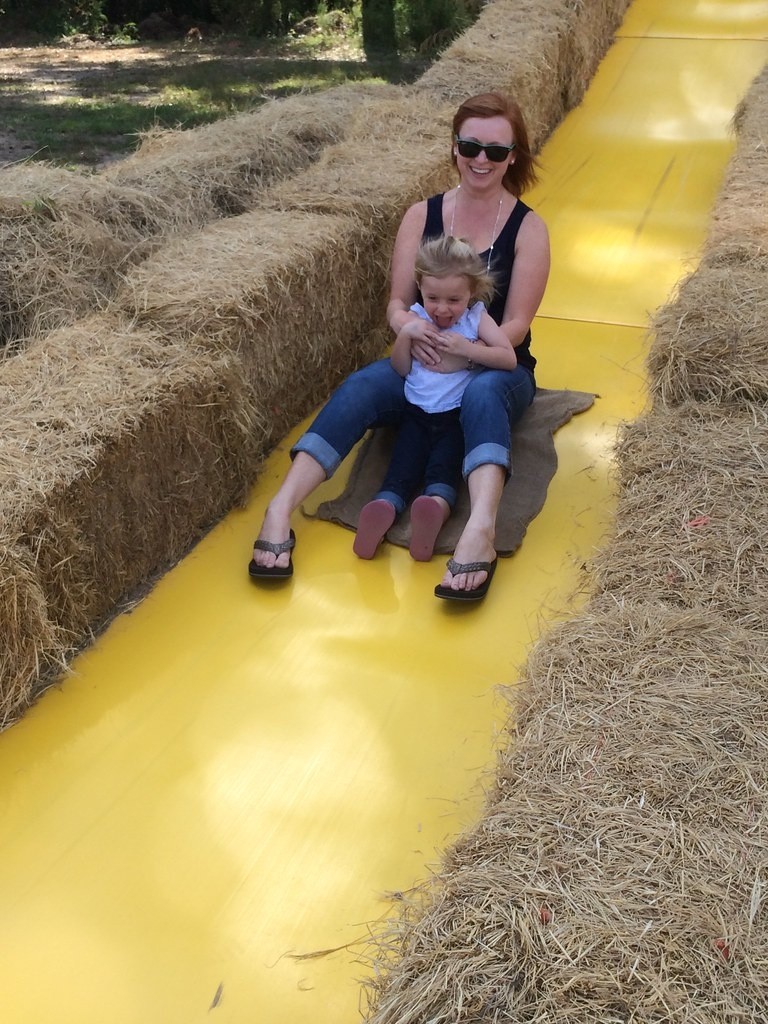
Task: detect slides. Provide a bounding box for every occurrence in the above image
[2,0,767,1024]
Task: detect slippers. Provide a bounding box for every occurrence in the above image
[434,556,497,601]
[248,527,295,578]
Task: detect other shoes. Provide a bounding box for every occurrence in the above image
[352,499,396,559]
[410,495,444,561]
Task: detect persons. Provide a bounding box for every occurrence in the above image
[352,235,518,562]
[249,91,550,604]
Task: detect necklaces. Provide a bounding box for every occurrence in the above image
[449,185,504,282]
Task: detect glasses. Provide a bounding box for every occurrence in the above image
[454,133,515,163]
